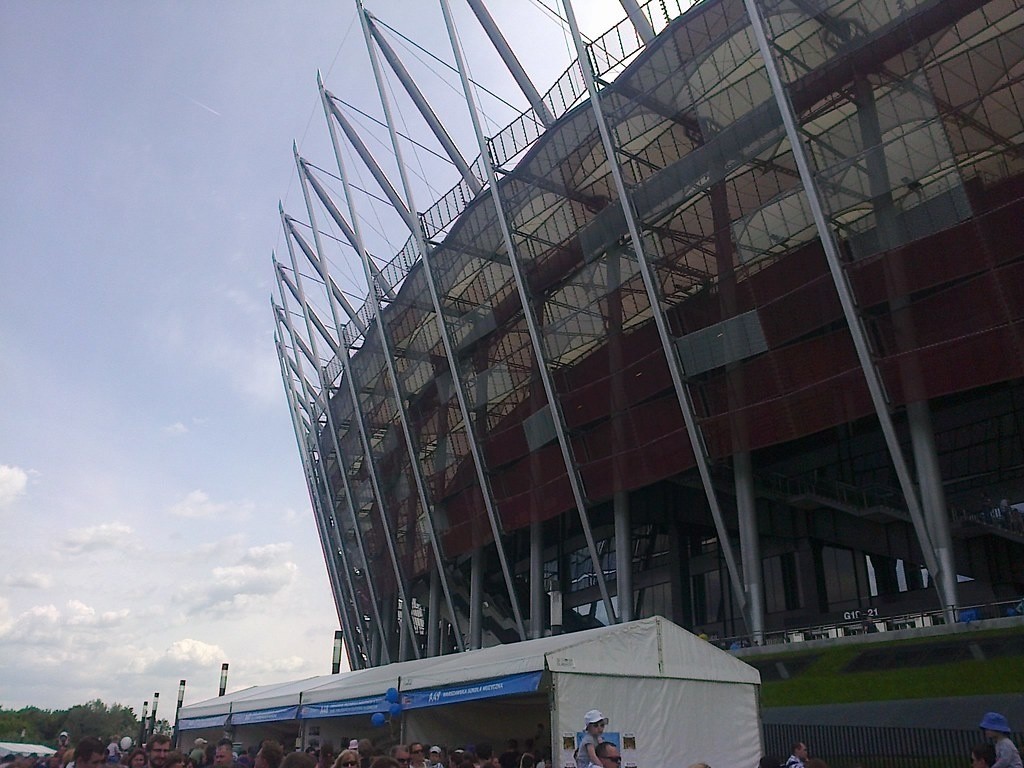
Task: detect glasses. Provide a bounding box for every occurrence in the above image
[803,749,807,752]
[85,758,107,765]
[589,723,604,728]
[599,756,621,763]
[411,749,424,755]
[970,757,977,764]
[342,761,357,767]
[396,758,412,763]
[150,749,170,753]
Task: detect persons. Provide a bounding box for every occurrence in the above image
[865,617,879,634]
[759,756,780,768]
[595,742,621,768]
[0,735,552,768]
[805,759,828,768]
[979,712,1023,768]
[775,629,818,644]
[710,637,766,650]
[786,742,808,768]
[972,743,997,768]
[577,710,609,768]
[980,491,1024,532]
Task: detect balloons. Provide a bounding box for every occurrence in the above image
[389,704,400,718]
[386,688,399,703]
[372,713,385,727]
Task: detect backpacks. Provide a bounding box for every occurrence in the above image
[780,761,798,768]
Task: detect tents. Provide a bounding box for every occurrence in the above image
[177,615,764,768]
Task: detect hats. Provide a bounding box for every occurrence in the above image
[348,739,358,750]
[583,710,609,731]
[429,745,441,754]
[194,737,208,745]
[978,711,1011,732]
[60,732,68,738]
